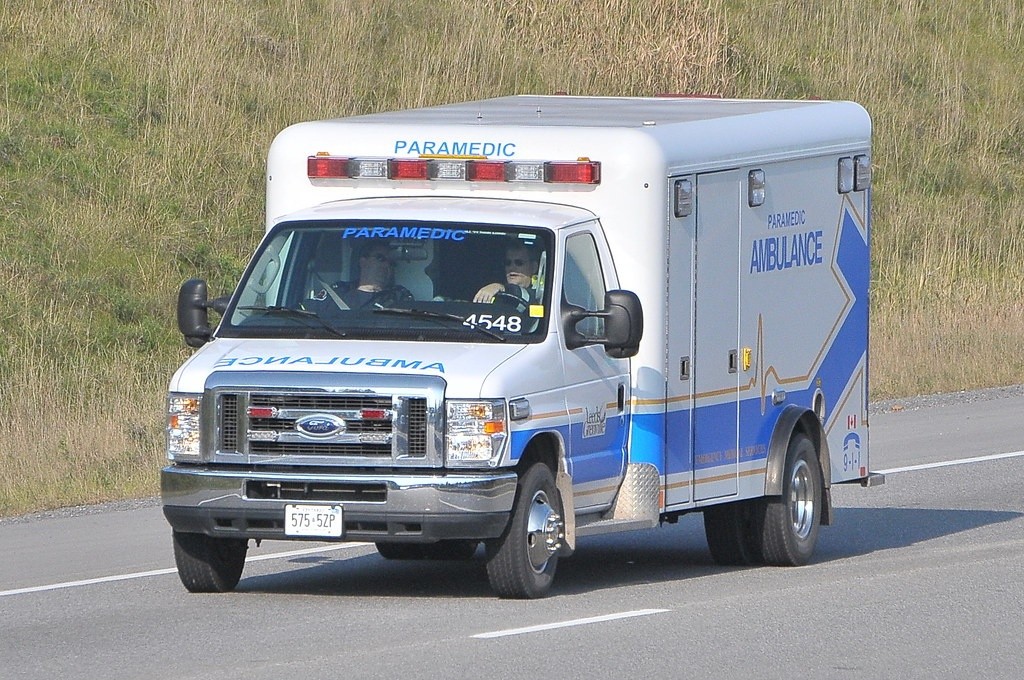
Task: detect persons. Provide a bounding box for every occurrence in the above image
[470,239,544,311]
[291,242,415,310]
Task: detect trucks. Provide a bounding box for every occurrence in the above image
[158,90,887,601]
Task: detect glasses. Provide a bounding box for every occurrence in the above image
[365,254,396,267]
[504,258,532,266]
